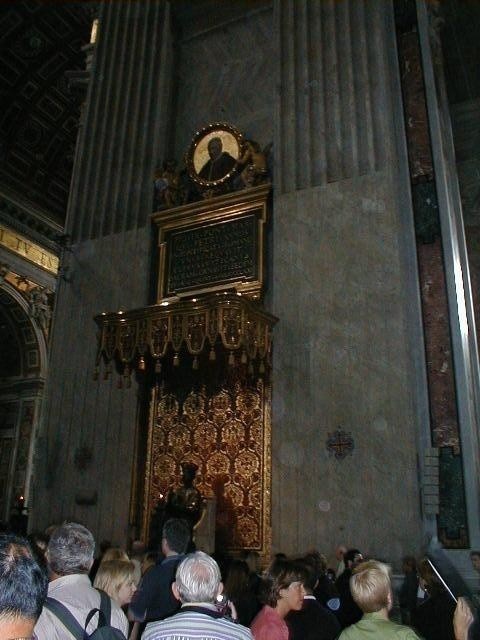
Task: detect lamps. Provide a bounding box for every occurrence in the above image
[16,496,28,515]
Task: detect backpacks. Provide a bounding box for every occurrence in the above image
[43,588,130,640]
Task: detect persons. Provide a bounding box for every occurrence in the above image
[148,464,202,552]
[2,519,480,640]
[237,141,268,188]
[162,158,186,206]
[197,138,237,179]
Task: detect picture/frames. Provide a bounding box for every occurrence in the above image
[184,123,243,187]
